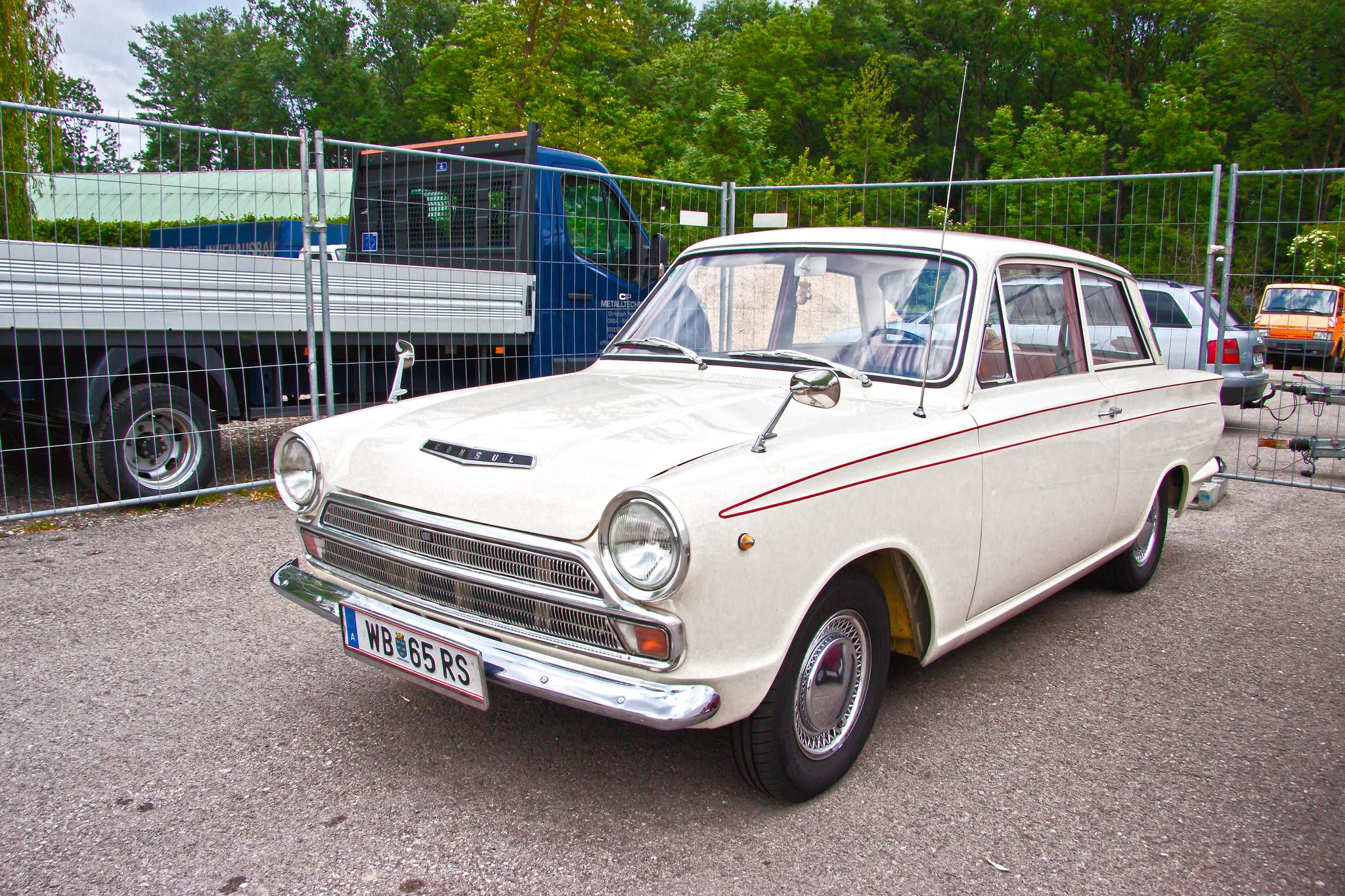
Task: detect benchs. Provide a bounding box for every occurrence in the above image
[862,340,1121,388]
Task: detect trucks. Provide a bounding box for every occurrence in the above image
[1250,284,1345,369]
[0,126,718,512]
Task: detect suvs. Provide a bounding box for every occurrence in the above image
[827,276,1271,406]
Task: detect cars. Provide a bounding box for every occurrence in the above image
[267,223,1230,801]
